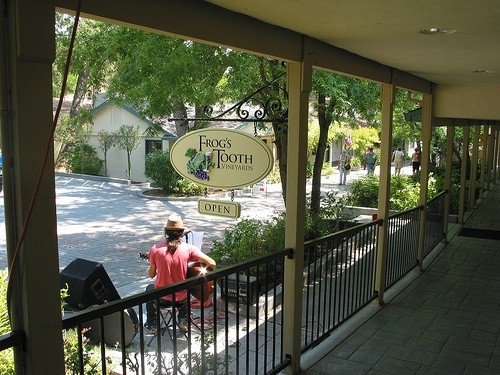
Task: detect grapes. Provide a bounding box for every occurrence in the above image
[185,148,215,181]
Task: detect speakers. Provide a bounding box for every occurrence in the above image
[59,258,121,310]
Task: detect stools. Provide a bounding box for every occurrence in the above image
[147,287,207,348]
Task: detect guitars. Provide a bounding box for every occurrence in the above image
[139,252,214,301]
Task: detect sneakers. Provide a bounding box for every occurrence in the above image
[178,324,189,332]
[144,323,158,334]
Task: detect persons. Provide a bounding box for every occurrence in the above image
[366,148,378,176]
[392,147,404,176]
[339,149,350,185]
[143,215,216,334]
[364,145,371,170]
[412,148,421,175]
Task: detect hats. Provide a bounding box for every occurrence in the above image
[164,215,187,231]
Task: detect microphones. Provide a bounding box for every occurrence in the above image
[180,230,191,238]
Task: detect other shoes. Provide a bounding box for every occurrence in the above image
[339,183,346,185]
